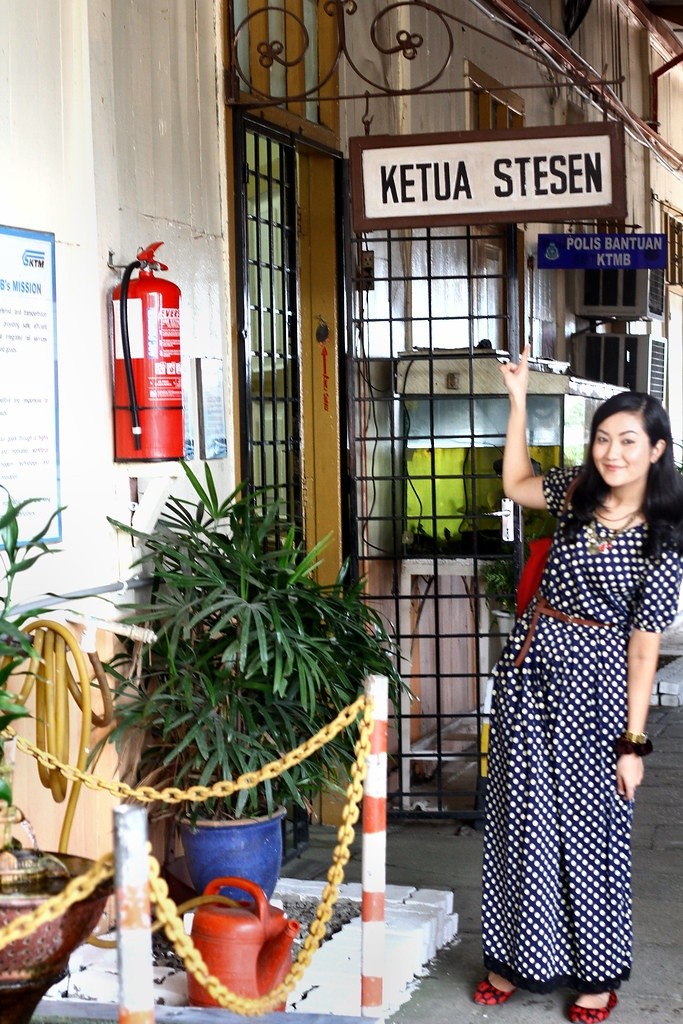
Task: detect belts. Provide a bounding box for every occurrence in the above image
[515,592,614,667]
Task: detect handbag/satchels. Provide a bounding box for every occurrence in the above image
[517,538,552,620]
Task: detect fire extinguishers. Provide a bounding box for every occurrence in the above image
[112,241,185,464]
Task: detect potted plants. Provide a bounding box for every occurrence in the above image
[106,469,415,900]
[0,490,118,1024]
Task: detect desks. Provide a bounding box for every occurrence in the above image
[398,560,492,813]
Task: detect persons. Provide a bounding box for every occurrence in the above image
[468,346,679,1023]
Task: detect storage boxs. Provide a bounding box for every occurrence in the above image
[399,392,608,556]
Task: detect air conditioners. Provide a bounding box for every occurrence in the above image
[585,332,667,406]
[571,267,667,320]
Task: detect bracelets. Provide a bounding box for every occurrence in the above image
[624,731,650,744]
[615,738,654,757]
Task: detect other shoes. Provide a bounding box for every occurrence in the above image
[568,990,618,1024]
[472,971,522,1006]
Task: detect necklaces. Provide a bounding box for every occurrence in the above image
[588,509,643,555]
